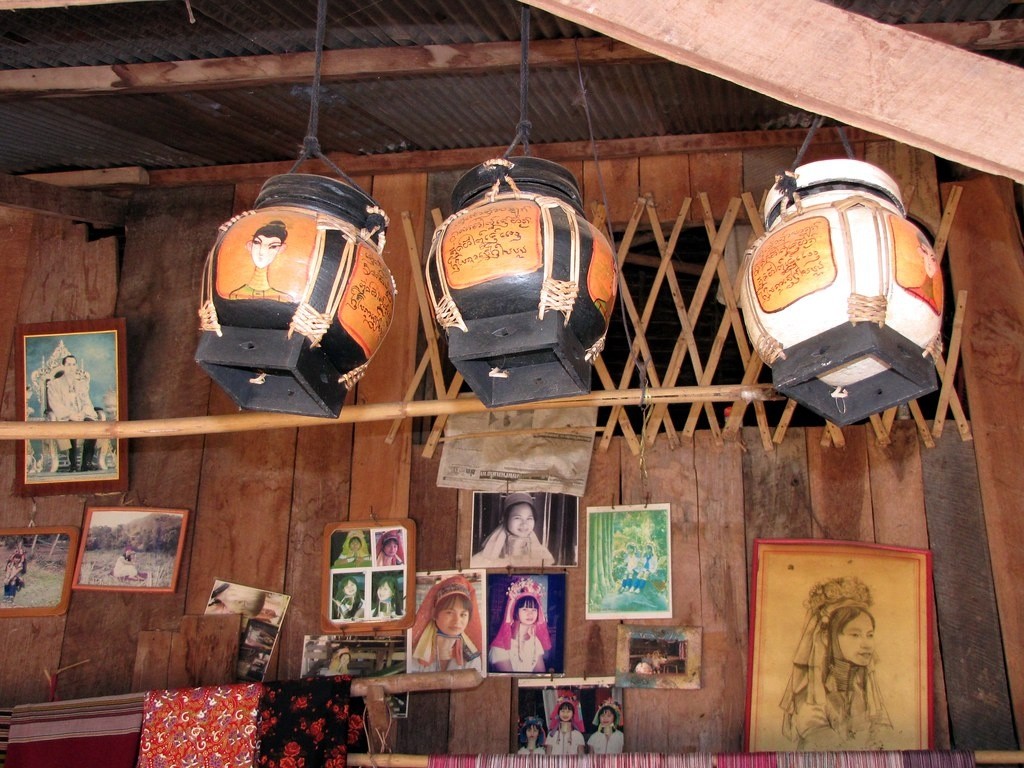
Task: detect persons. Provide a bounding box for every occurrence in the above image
[2,548,28,600]
[413,574,482,673]
[330,647,350,674]
[332,573,364,618]
[113,545,144,581]
[641,649,668,674]
[488,576,552,673]
[371,575,403,617]
[377,530,403,566]
[472,492,556,566]
[44,355,100,471]
[620,543,657,595]
[246,627,274,649]
[333,530,371,568]
[515,693,624,754]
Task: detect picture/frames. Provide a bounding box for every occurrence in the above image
[12,317,129,497]
[320,519,415,634]
[0,525,81,618]
[72,505,190,594]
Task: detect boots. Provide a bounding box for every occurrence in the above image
[81,439,98,471]
[69,440,78,472]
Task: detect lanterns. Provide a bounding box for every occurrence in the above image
[192,172,397,418]
[740,157,945,429]
[426,157,621,408]
